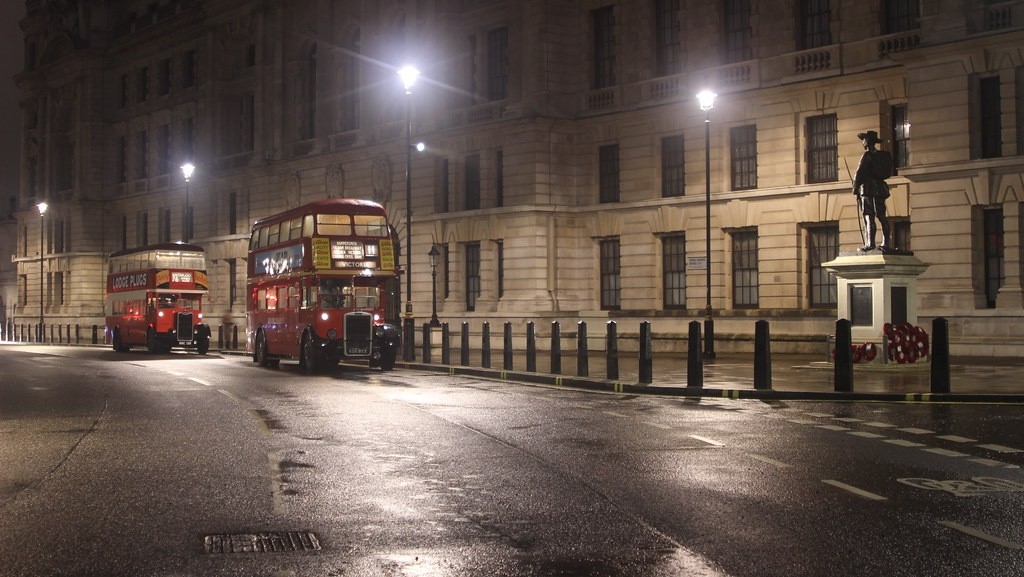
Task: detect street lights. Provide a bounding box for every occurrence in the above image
[180,161,196,243]
[35,202,49,342]
[395,62,421,360]
[695,87,719,358]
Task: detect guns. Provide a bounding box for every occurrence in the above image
[843,156,868,246]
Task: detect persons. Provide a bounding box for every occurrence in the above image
[852,131,893,252]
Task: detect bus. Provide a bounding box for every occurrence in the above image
[104,243,211,354]
[244,198,405,372]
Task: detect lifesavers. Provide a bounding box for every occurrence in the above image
[883,323,929,364]
[861,342,877,361]
[851,345,861,363]
[832,348,835,360]
